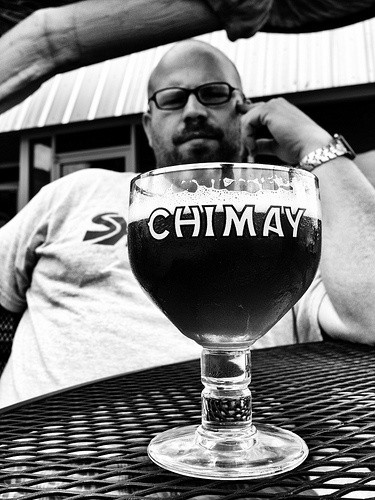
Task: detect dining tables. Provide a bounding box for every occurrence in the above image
[1,339,375,500]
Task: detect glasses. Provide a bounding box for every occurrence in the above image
[148,82,246,110]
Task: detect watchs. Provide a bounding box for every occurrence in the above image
[297,133,358,172]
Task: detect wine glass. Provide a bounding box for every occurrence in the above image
[127,162,323,482]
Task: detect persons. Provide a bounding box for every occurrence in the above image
[1,41,375,408]
